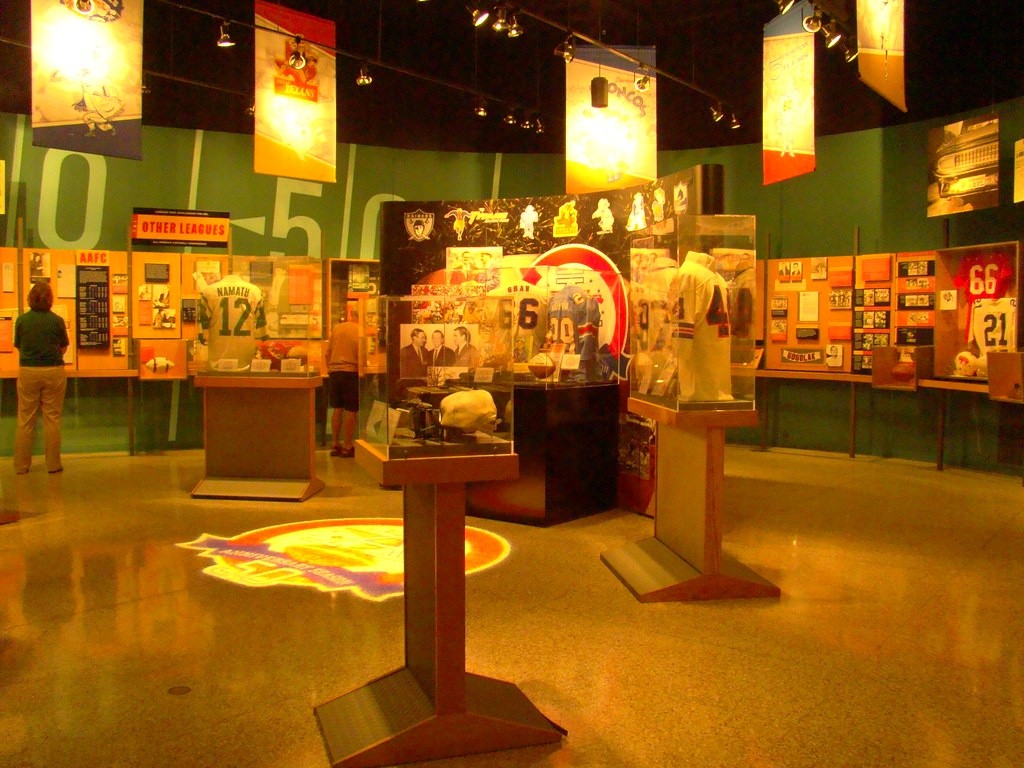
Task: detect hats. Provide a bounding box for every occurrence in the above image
[440,390,502,430]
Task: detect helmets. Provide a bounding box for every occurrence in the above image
[955,352,977,375]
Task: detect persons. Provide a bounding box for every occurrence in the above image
[401,328,478,379]
[514,284,601,383]
[771,261,929,369]
[325,303,367,457]
[200,275,266,372]
[449,251,500,292]
[640,252,738,402]
[513,338,529,363]
[13,281,69,473]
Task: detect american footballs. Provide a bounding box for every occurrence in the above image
[287,347,310,365]
[146,356,174,373]
[890,364,915,383]
[528,353,556,378]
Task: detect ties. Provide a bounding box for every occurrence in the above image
[418,348,421,359]
[435,349,437,361]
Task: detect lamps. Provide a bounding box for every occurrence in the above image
[503,109,516,124]
[475,100,489,116]
[508,15,523,37]
[492,9,510,32]
[635,72,650,92]
[803,6,823,33]
[218,21,236,47]
[536,117,546,134]
[520,117,534,128]
[775,0,794,15]
[356,64,372,85]
[591,0,608,108]
[288,37,306,70]
[844,46,858,63]
[730,113,740,129]
[710,103,723,122]
[820,20,842,49]
[554,37,575,63]
[466,5,489,27]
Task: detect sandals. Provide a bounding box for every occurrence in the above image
[331,445,354,457]
